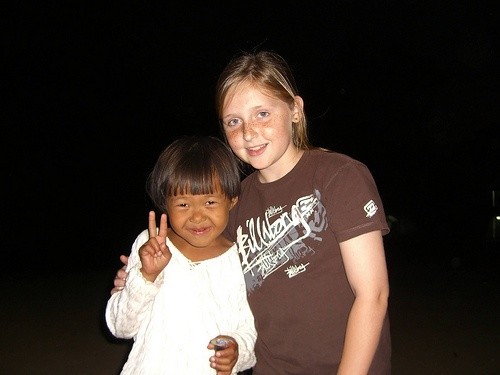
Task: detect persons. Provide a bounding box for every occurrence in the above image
[110,51,391,375]
[104,134,259,375]
